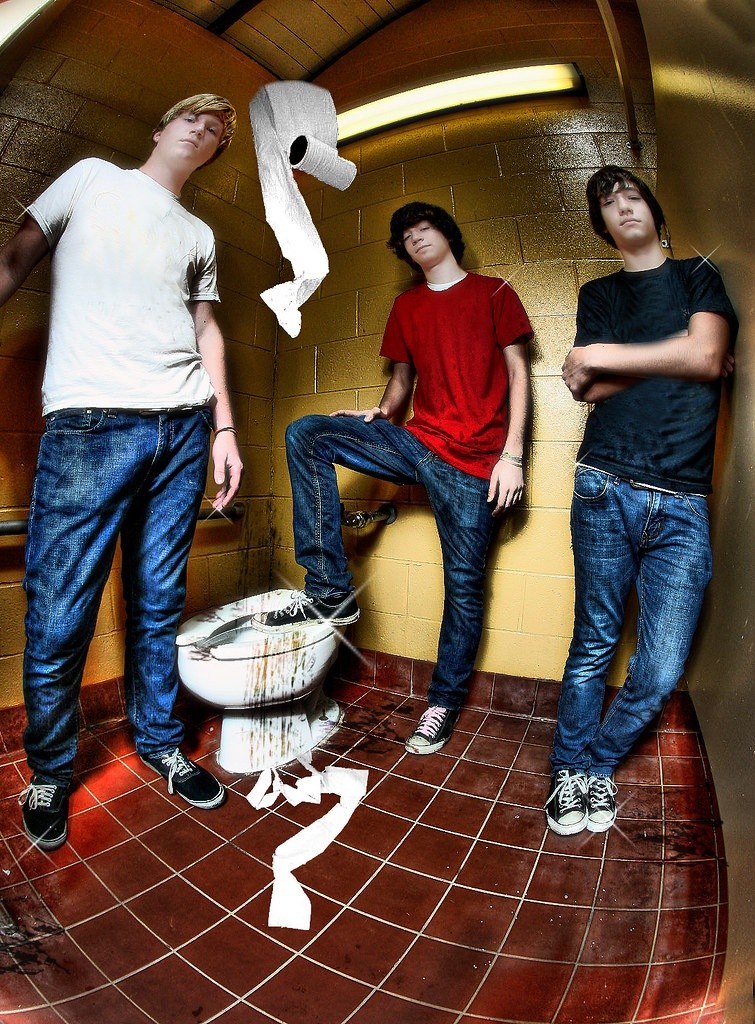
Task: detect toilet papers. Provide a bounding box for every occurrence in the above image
[252,79,357,340]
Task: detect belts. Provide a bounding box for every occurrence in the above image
[124,405,206,417]
[621,477,650,490]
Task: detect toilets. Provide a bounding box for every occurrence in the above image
[179,584,346,772]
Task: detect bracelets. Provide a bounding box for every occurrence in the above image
[500,450,522,468]
[215,427,238,439]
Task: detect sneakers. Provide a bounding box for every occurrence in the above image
[252,588,361,634]
[586,771,618,832]
[18,773,68,850]
[543,768,588,836]
[405,705,461,754]
[139,745,226,810]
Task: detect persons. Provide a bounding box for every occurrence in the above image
[545,164,738,835]
[250,201,534,757]
[1,94,244,844]
